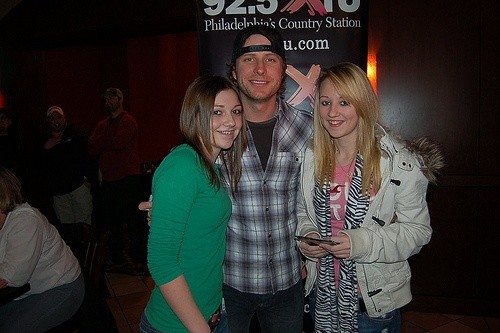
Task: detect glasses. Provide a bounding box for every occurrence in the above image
[48,116,61,119]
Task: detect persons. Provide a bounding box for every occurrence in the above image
[138,25,314,333]
[293,62,447,333]
[138,75,249,333]
[0,88,138,333]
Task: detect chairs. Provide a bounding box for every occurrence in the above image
[53,220,110,333]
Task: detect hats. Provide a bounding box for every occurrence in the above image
[100,87,123,100]
[47,105,64,117]
[232,25,286,63]
